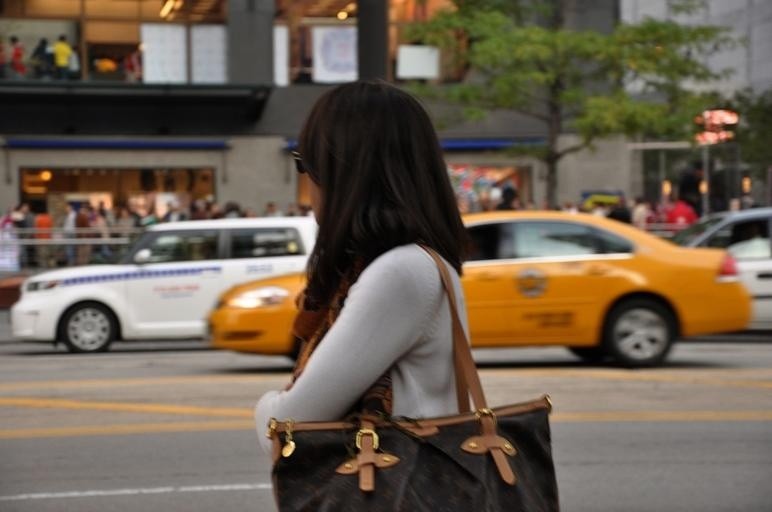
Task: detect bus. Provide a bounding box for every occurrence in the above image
[682,159,753,214]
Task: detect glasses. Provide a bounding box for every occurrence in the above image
[291,149,305,174]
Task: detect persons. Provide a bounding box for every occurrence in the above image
[254,81,469,453]
[0,33,142,83]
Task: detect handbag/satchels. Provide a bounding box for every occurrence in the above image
[263,240,562,512]
